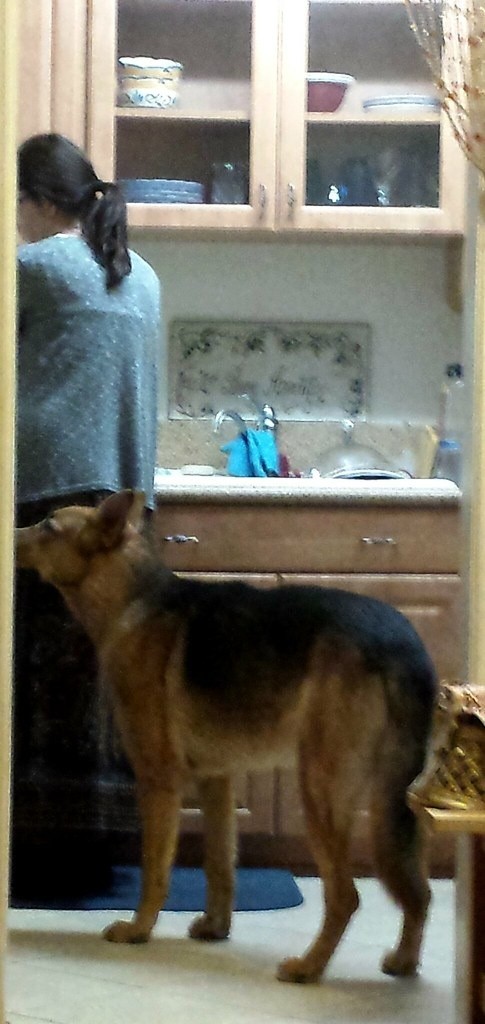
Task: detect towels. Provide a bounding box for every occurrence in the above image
[220,429,279,477]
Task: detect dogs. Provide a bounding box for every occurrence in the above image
[15,489,436,984]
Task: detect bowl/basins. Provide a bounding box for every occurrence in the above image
[118,56,183,107]
[306,82,346,112]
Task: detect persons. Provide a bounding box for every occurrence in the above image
[7,133,160,877]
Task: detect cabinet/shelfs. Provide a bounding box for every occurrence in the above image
[86,0,473,233]
[153,503,467,865]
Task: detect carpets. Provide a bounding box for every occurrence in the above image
[15,856,308,912]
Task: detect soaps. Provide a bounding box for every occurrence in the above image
[181,465,216,475]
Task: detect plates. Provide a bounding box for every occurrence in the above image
[308,70,357,85]
[362,95,443,115]
[118,177,205,204]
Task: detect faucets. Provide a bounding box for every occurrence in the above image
[211,393,279,446]
